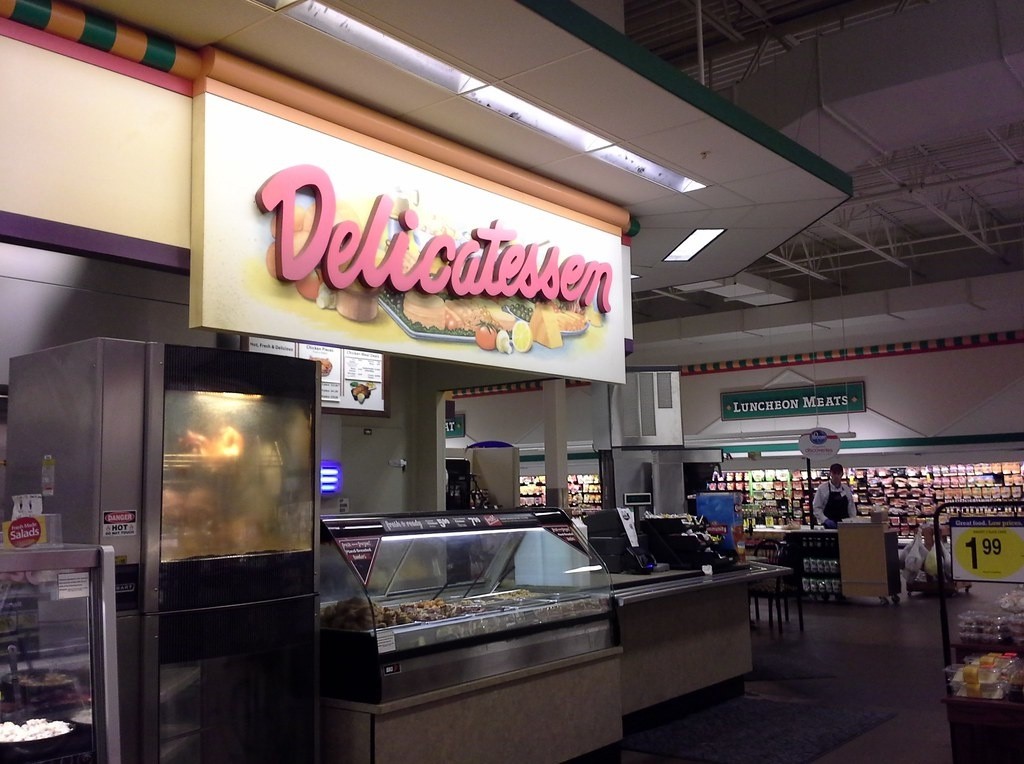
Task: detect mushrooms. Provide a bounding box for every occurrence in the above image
[495,330,512,354]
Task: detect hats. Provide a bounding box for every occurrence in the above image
[830,463,842,470]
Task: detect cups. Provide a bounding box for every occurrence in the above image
[765,516,773,528]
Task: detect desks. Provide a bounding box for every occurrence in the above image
[751,524,824,539]
[784,530,841,603]
[746,554,767,565]
[838,521,902,604]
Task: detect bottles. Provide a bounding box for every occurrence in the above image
[802,537,837,549]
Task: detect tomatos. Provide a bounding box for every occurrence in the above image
[476,324,498,350]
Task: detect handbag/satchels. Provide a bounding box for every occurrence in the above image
[899,530,952,575]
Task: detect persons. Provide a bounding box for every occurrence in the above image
[812,464,857,528]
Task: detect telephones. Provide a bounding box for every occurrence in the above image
[625,545,657,573]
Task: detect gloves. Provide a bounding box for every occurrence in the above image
[824,519,836,527]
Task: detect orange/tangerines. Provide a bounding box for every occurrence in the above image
[512,320,533,353]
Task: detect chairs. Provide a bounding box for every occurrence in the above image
[747,538,789,622]
[751,544,804,636]
